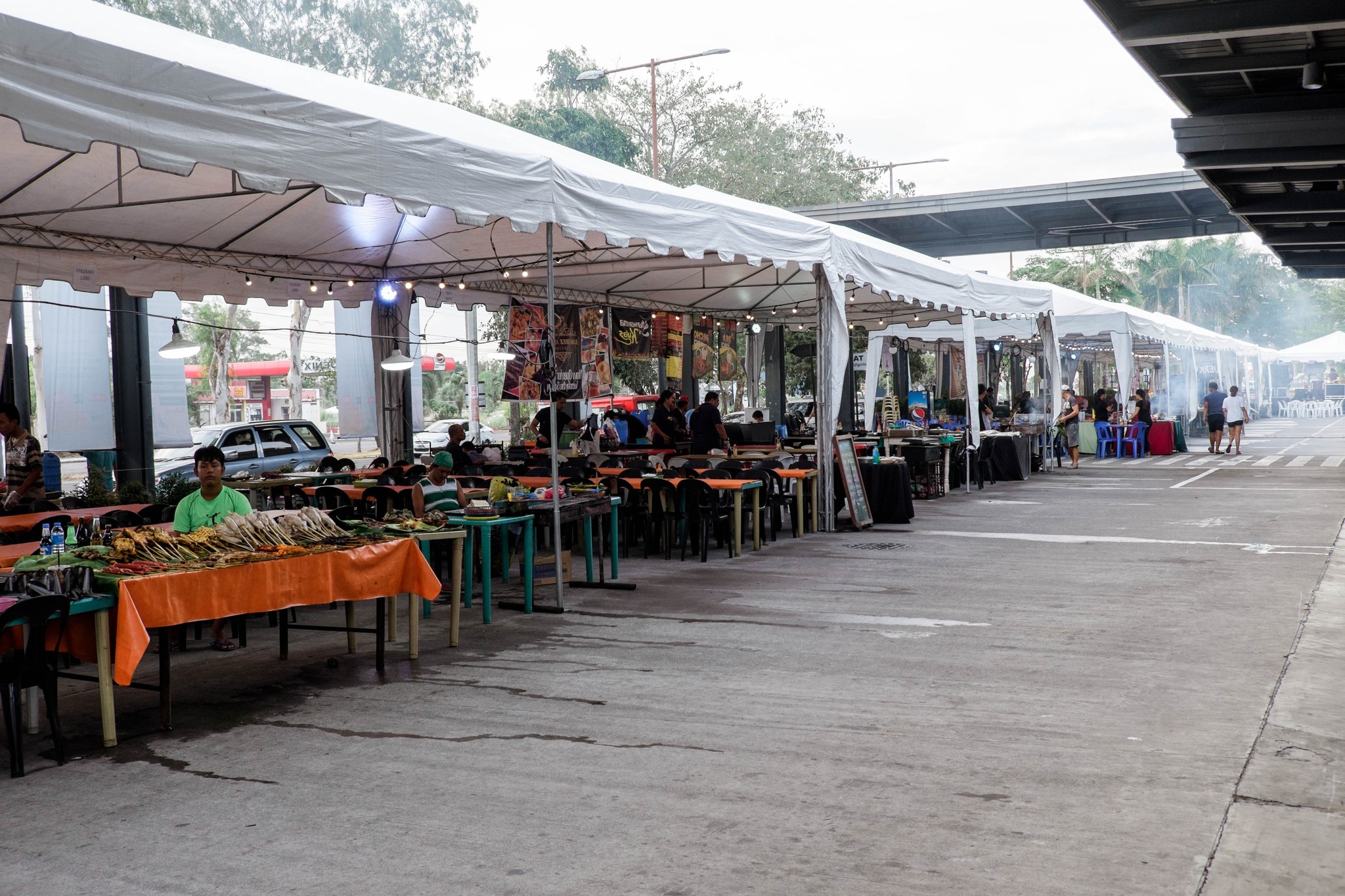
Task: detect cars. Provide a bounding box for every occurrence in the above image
[413,419,494,457]
[786,395,816,431]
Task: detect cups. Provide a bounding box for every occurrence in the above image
[506,487,515,498]
[515,487,524,494]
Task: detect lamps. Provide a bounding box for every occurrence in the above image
[158,320,201,359]
[1067,354,1076,360]
[742,324,761,336]
[488,341,515,360]
[379,284,397,306]
[1155,363,1162,370]
[994,342,1000,351]
[381,338,413,371]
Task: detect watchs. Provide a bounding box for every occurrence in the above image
[724,437,729,441]
[538,434,542,438]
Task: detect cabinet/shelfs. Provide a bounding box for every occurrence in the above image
[967,434,1031,480]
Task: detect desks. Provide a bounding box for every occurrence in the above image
[670,436,914,565]
[1077,420,1189,455]
[221,447,675,624]
[0,504,469,747]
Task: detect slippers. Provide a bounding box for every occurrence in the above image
[1216,451,1225,454]
[209,640,237,650]
[441,582,452,591]
[1236,451,1242,454]
[1208,447,1214,453]
[153,643,180,654]
[460,581,476,589]
[1226,444,1232,453]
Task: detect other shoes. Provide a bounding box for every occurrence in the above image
[1067,464,1078,470]
[1144,453,1151,457]
[1137,453,1141,455]
[1105,451,1116,455]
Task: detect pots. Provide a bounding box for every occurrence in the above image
[558,428,579,448]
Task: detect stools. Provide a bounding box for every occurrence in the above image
[873,394,901,429]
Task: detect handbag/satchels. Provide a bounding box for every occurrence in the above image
[602,417,621,446]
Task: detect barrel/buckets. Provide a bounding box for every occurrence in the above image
[42,450,61,491]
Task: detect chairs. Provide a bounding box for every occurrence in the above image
[1277,398,1344,419]
[0,594,71,778]
[1017,419,1147,468]
[784,410,818,437]
[895,416,997,489]
[314,447,845,585]
[273,433,290,444]
[0,485,313,650]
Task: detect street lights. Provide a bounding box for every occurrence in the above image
[1055,245,1120,296]
[576,49,731,181]
[850,158,948,200]
[1188,283,1217,324]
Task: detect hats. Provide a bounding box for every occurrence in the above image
[433,451,453,469]
[1062,384,1071,391]
[679,395,689,403]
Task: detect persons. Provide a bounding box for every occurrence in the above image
[751,410,764,423]
[603,410,647,444]
[1020,392,1035,414]
[1112,389,1152,457]
[1058,384,1079,469]
[529,391,588,450]
[650,390,730,455]
[806,405,815,424]
[153,445,251,654]
[0,401,45,511]
[978,384,995,431]
[411,424,489,593]
[1328,368,1339,384]
[67,451,117,493]
[1093,388,1116,455]
[1203,382,1249,454]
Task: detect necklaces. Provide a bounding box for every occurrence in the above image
[436,480,444,493]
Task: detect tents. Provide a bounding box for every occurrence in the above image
[1278,331,1345,363]
[831,221,1278,422]
[0,0,831,605]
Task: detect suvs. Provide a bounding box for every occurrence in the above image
[78,419,333,498]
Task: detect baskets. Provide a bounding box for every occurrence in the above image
[463,505,499,517]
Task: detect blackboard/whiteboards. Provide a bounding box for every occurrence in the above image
[834,433,874,527]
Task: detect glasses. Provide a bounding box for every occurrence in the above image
[711,398,721,404]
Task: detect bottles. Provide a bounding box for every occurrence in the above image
[90,515,103,545]
[921,421,925,429]
[803,423,806,434]
[925,421,929,430]
[103,524,114,546]
[656,468,662,476]
[836,422,843,435]
[872,446,880,464]
[51,523,65,554]
[733,444,738,457]
[65,522,78,552]
[75,516,89,548]
[799,424,804,437]
[776,437,781,451]
[727,447,732,457]
[39,524,53,555]
[781,437,785,451]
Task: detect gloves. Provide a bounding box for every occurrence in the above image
[723,440,731,449]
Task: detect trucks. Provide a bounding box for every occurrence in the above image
[591,394,658,443]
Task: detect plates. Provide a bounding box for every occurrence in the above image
[463,514,500,520]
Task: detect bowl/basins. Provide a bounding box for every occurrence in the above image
[497,499,529,516]
[642,476,663,480]
[524,440,537,446]
